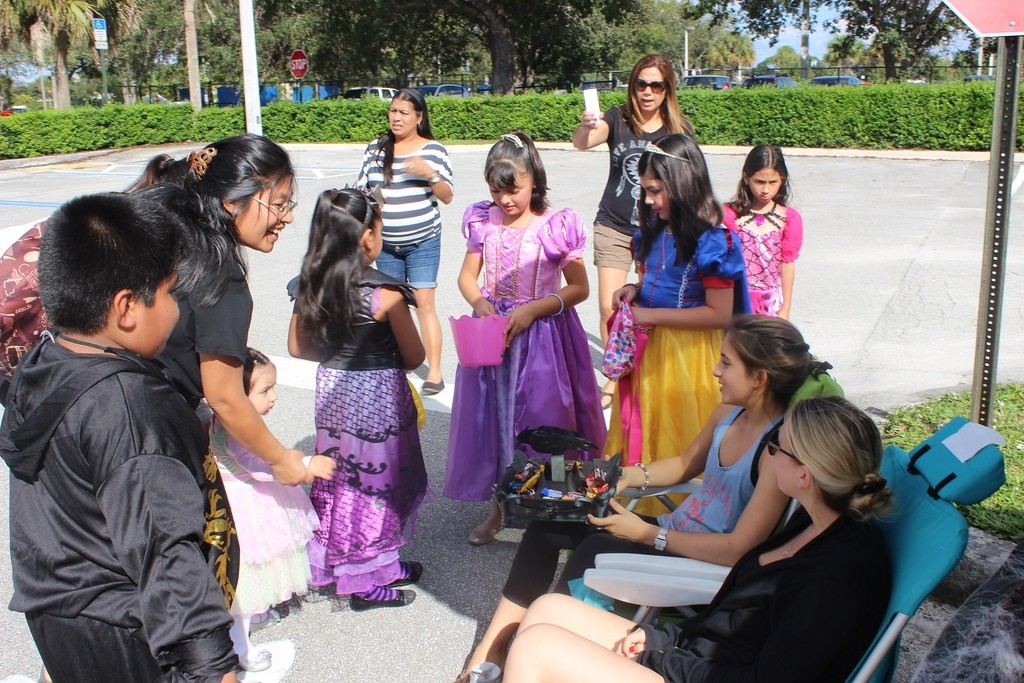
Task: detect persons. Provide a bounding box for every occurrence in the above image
[501,396,894,683]
[0,192,236,682]
[601,131,751,518]
[358,88,446,394]
[574,54,694,410]
[123,133,315,610]
[454,314,814,683]
[287,182,436,614]
[443,133,608,545]
[720,145,804,322]
[208,347,320,671]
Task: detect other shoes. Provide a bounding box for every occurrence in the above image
[238,648,271,673]
[457,662,503,683]
[250,612,269,624]
[420,380,444,397]
[468,523,504,546]
[377,561,423,587]
[289,593,302,609]
[349,587,416,611]
[275,602,290,619]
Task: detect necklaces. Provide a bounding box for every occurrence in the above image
[782,544,796,555]
[662,229,677,269]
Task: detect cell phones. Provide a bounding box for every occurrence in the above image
[584,88,601,126]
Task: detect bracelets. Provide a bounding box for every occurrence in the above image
[635,462,649,492]
[544,294,564,316]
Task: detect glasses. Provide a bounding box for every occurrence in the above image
[635,79,667,94]
[768,430,804,466]
[250,195,298,221]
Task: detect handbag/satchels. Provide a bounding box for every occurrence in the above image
[407,379,427,430]
[600,296,655,382]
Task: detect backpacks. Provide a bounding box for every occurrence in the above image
[0,222,53,379]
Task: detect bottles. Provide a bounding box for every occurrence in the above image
[583,89,602,124]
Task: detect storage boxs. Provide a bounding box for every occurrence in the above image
[492,449,622,527]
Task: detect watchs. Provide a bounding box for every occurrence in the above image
[655,527,669,550]
[427,170,441,183]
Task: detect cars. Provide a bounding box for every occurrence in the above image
[964,74,995,82]
[12,105,26,113]
[809,76,859,85]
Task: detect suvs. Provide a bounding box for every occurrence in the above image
[742,74,795,87]
[414,82,470,98]
[678,75,731,90]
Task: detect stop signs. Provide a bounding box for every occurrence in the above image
[289,50,308,80]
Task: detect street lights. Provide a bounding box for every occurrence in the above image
[684,25,694,75]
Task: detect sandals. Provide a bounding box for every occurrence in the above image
[599,390,614,410]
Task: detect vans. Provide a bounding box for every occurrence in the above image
[344,88,398,100]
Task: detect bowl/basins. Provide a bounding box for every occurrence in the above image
[448,314,511,367]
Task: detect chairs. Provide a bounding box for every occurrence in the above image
[589,414,1008,683]
[593,370,848,580]
